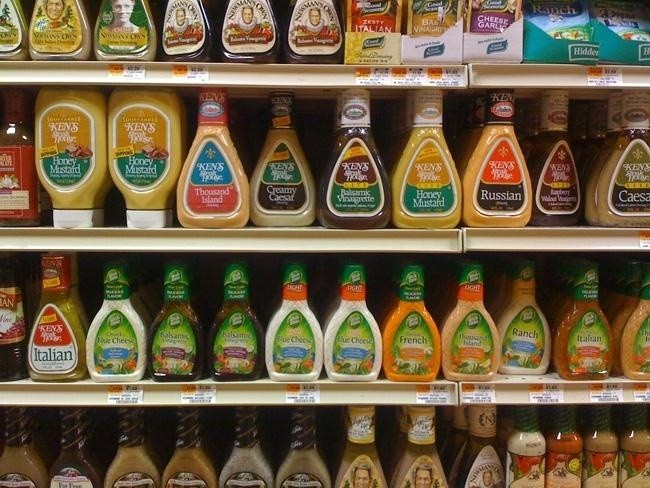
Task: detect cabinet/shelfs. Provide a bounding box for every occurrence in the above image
[460,59,650,406]
[0,60,471,409]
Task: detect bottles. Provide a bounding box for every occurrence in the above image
[283,0,344,64]
[0,249,650,382]
[0,82,650,229]
[162,0,212,61]
[219,0,280,63]
[0,0,29,61]
[29,0,91,62]
[0,403,650,488]
[92,1,157,62]
